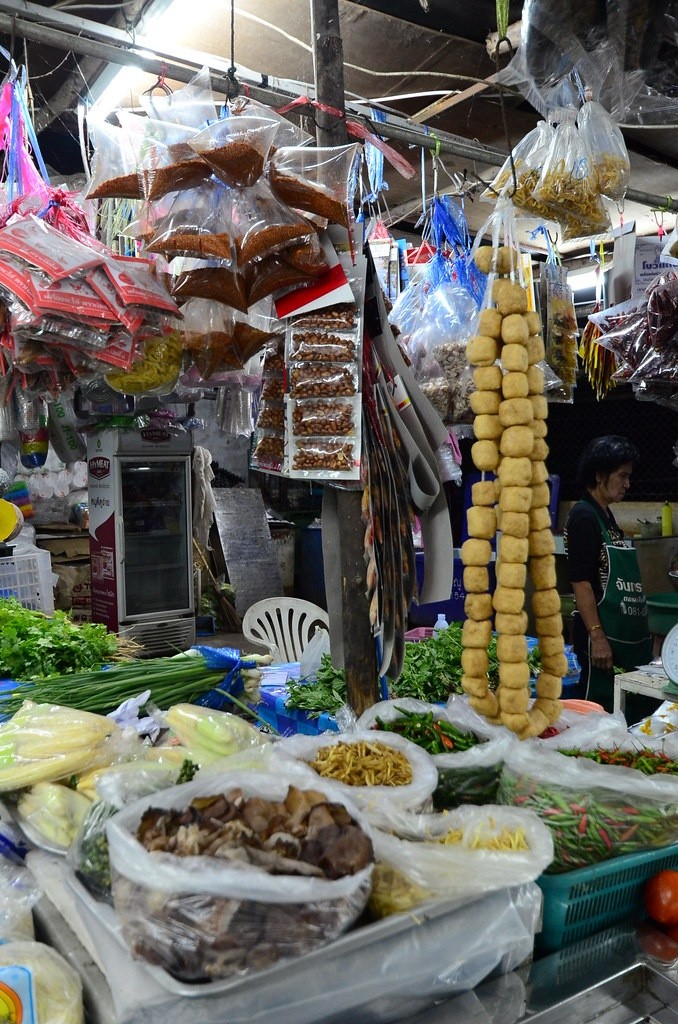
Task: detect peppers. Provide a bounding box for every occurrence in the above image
[378,705,677,870]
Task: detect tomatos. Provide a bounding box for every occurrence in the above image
[643,871,678,925]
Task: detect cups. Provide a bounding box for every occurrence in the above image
[656,516,662,536]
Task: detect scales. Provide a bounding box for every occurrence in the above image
[660,568,678,697]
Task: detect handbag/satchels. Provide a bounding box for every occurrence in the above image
[299,625,331,683]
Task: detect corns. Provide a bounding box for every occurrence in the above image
[0,703,260,848]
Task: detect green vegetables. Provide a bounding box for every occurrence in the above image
[0,596,139,679]
[288,626,544,716]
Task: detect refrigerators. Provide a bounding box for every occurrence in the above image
[87,424,196,661]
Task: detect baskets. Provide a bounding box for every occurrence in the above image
[536,844,678,950]
[404,627,434,642]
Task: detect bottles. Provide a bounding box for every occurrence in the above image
[661,499,673,536]
[433,614,449,642]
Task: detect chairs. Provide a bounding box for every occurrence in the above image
[243,597,330,665]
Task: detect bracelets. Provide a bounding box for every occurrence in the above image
[586,624,602,632]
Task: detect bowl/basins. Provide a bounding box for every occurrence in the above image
[637,523,661,538]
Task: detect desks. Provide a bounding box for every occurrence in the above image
[613,670,678,719]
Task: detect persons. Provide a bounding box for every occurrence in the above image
[563,435,652,724]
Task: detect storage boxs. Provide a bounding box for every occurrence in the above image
[0,543,53,618]
[39,538,93,625]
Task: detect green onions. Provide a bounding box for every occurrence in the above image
[0,646,274,719]
